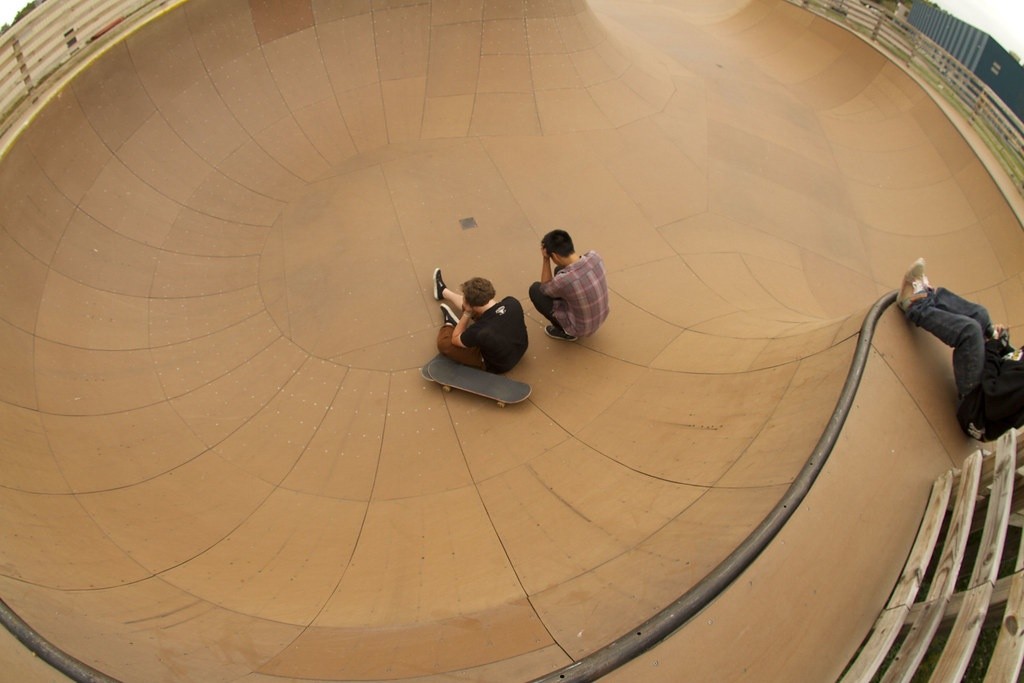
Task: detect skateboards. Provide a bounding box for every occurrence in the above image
[421,353,447,381]
[428,359,533,408]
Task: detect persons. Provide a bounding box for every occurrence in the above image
[529,229,610,342]
[897,257,1024,442]
[433,267,529,375]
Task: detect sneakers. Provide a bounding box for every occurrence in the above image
[440,305,461,329]
[432,268,448,301]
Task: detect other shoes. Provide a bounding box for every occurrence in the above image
[544,325,578,343]
[896,258,934,313]
